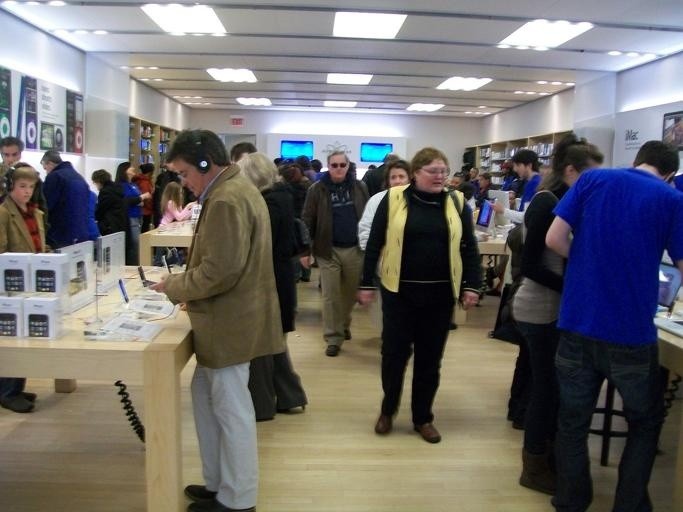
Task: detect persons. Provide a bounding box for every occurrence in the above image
[451,159,492,214]
[357,148,482,443]
[302,152,370,356]
[87,191,101,242]
[167,130,285,512]
[41,151,88,249]
[500,161,519,191]
[155,181,198,265]
[0,167,36,412]
[362,154,399,197]
[231,142,258,163]
[93,169,133,252]
[487,149,542,226]
[0,138,49,237]
[514,133,603,496]
[237,152,308,422]
[116,163,152,265]
[547,141,683,512]
[134,163,151,231]
[507,325,532,430]
[358,160,411,278]
[274,157,329,189]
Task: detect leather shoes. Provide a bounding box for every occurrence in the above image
[374,410,399,434]
[344,329,351,340]
[414,422,441,443]
[184,484,217,501]
[326,345,340,356]
[187,499,256,512]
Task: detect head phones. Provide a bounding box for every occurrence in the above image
[190,129,212,174]
[2,163,30,191]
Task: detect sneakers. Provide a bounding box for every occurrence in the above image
[512,420,527,429]
[0,395,34,412]
[20,391,36,400]
[507,411,513,420]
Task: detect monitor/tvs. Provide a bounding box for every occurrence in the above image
[476,197,498,239]
[281,140,315,161]
[359,143,393,163]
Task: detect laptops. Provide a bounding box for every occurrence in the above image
[487,190,509,225]
[656,262,683,312]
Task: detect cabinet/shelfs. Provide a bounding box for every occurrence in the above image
[462,129,572,184]
[127,115,183,183]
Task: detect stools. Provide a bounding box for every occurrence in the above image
[588,380,630,467]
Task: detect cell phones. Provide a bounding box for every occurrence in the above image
[77,262,86,283]
[162,255,172,275]
[144,280,157,286]
[35,271,54,291]
[0,312,17,335]
[104,247,110,273]
[118,279,129,304]
[138,266,146,287]
[29,314,48,337]
[4,269,24,291]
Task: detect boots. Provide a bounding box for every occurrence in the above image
[519,448,561,496]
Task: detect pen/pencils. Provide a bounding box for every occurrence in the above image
[118,280,128,303]
[164,255,172,274]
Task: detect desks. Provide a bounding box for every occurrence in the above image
[137,219,197,264]
[0,264,194,512]
[471,232,514,299]
[655,311,682,378]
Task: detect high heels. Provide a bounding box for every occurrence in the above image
[277,399,307,412]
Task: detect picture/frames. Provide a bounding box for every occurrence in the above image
[661,111,682,150]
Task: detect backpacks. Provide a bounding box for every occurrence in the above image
[271,192,313,262]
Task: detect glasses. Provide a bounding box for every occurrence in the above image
[331,162,347,168]
[421,168,450,175]
